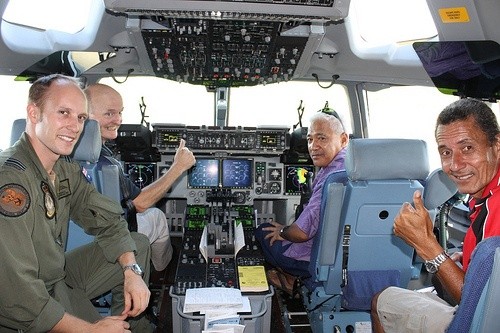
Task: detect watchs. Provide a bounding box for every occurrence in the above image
[425,251,450,274]
[122,263,144,276]
[279,225,288,238]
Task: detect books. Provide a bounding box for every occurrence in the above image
[238,265,270,293]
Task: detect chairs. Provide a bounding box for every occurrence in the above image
[444,236,500,332]
[10,117,121,314]
[276,137,430,333]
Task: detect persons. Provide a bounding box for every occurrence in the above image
[253,112,348,279]
[370,99,500,333]
[0,74,157,333]
[82,82,198,272]
